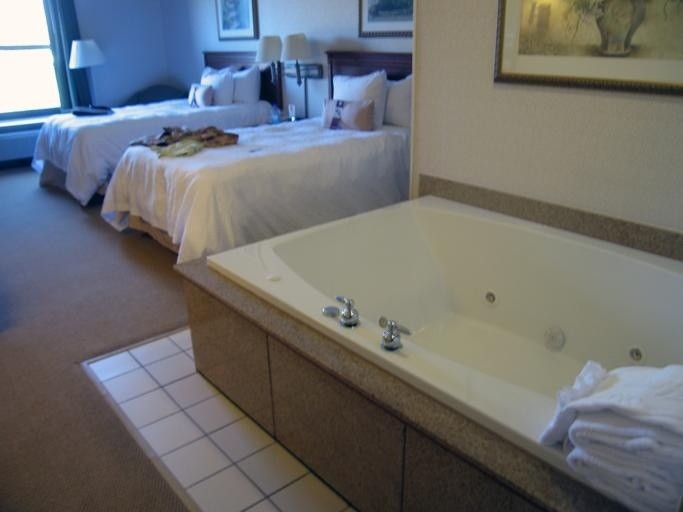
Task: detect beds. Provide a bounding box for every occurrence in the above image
[38,49,281,210]
[97,49,412,261]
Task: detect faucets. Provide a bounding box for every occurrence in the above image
[335,295,360,327]
[378,316,410,351]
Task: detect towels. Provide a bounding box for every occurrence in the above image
[537,355,682,512]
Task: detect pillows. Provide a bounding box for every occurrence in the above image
[319,68,413,132]
[188,64,262,110]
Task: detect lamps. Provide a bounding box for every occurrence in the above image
[257,34,292,84]
[68,38,105,105]
[283,33,312,86]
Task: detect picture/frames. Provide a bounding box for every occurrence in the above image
[215,0,259,42]
[491,0,682,102]
[358,0,412,39]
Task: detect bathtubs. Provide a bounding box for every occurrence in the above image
[172,198,683,512]
